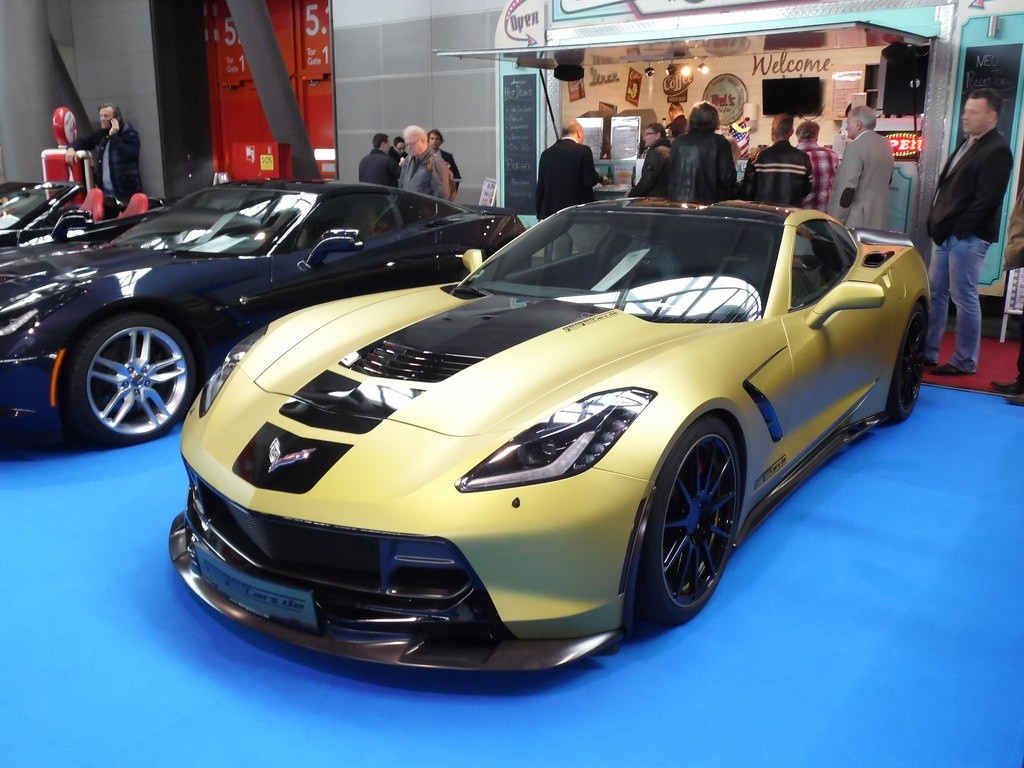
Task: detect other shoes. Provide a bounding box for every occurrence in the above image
[930,360,977,377]
[924,357,938,367]
[991,377,1024,394]
[1004,393,1024,406]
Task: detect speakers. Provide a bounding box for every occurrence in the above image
[876,42,930,116]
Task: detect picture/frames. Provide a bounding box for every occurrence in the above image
[478,177,497,207]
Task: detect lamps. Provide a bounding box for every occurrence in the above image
[645,61,655,77]
[666,60,677,77]
[697,56,709,75]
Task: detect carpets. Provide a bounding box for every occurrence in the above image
[921,331,1022,396]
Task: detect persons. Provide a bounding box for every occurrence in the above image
[536,120,609,222]
[827,105,895,230]
[668,100,813,208]
[924,87,1014,376]
[359,124,461,202]
[65,102,143,205]
[624,123,672,198]
[795,120,839,214]
[991,137,1024,406]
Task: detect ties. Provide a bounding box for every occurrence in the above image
[411,159,420,178]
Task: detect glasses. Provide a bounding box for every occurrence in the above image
[641,132,655,135]
[404,139,420,147]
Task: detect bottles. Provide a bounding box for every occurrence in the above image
[661,118,666,129]
[631,166,636,187]
[607,166,613,185]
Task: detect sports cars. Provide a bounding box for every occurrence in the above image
[0,175,527,450]
[0,182,169,252]
[167,197,930,669]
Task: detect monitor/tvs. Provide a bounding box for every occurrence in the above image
[762,77,821,115]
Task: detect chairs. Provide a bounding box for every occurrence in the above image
[612,229,687,290]
[79,188,104,222]
[119,193,149,219]
[270,210,314,250]
[340,202,376,240]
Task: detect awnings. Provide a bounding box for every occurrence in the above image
[432,20,938,174]
